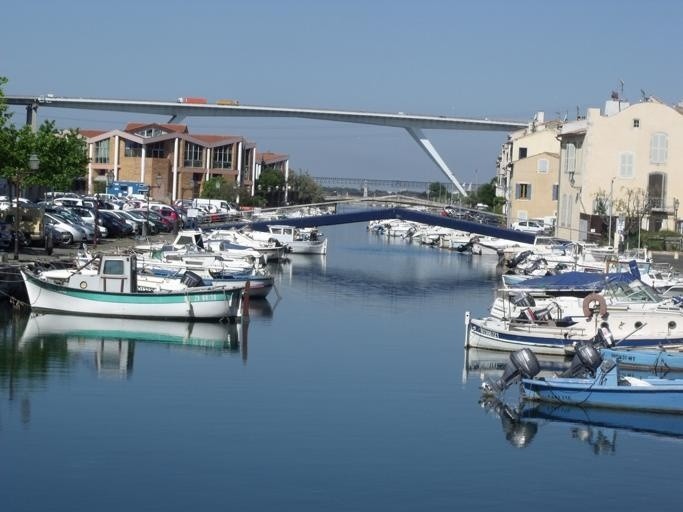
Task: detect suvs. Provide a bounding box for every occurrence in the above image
[513,222,545,234]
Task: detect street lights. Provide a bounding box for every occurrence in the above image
[11,151,39,261]
[140,172,161,240]
[230,183,291,208]
[90,169,113,250]
[674,198,679,236]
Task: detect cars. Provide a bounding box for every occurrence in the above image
[0,192,239,249]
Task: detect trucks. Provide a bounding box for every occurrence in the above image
[215,98,239,107]
[510,215,556,231]
[175,98,207,104]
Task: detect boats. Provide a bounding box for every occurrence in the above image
[498,236,683,323]
[460,308,680,370]
[18,310,239,377]
[253,224,328,254]
[72,225,286,298]
[21,255,245,319]
[366,204,524,257]
[477,350,682,414]
[462,348,569,385]
[479,390,681,454]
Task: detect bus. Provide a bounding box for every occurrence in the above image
[105,181,148,196]
